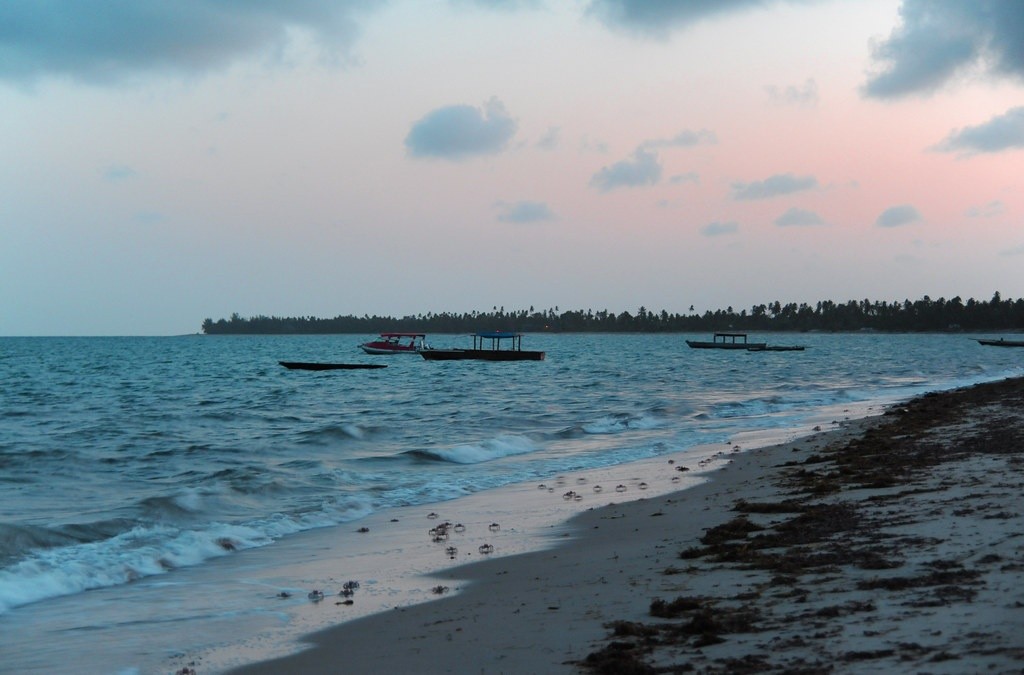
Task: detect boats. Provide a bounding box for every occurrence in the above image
[277,360,388,370]
[357,332,434,354]
[746,344,805,351]
[977,337,1024,346]
[418,345,465,361]
[452,329,545,361]
[685,332,766,349]
